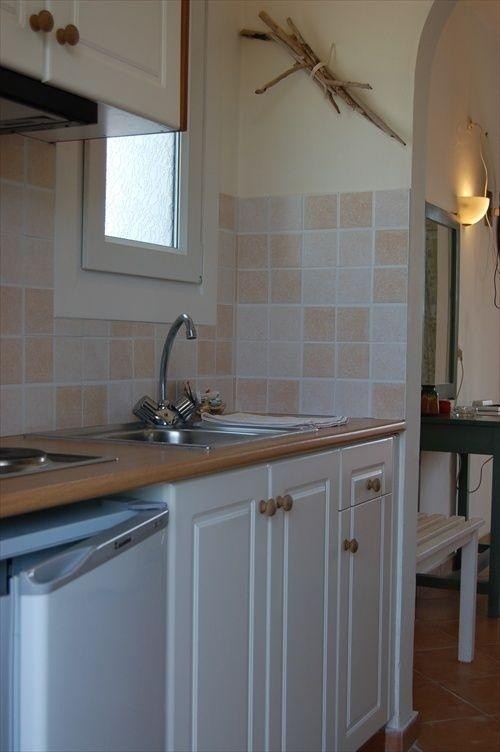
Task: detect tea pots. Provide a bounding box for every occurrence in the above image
[423,198,461,402]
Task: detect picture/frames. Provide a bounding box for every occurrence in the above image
[415,410,500,621]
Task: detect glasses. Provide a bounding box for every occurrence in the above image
[457,194,489,228]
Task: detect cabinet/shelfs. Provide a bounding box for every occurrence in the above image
[420,384,440,417]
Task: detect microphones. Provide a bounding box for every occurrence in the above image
[414,507,484,664]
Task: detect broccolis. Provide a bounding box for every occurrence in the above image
[1,494,177,752]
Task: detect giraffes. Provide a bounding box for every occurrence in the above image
[0,445,118,481]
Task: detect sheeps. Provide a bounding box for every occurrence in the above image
[159,314,197,400]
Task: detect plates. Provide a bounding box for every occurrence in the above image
[2,0,191,143]
[338,431,400,751]
[125,450,340,750]
